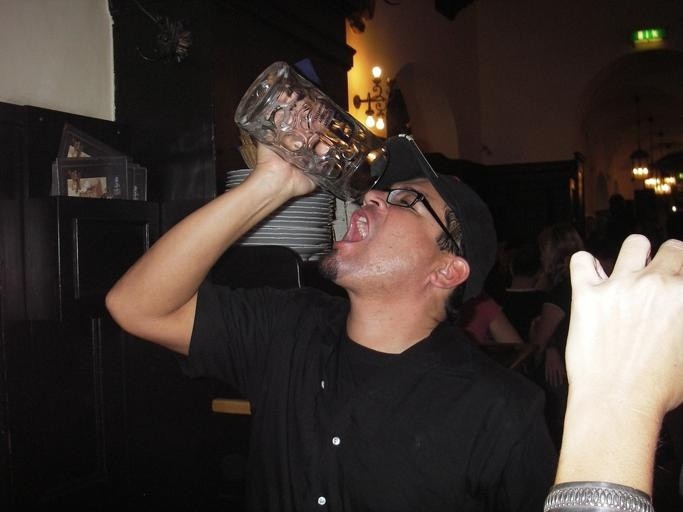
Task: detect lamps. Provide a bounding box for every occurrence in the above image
[629,52,663,188]
[351,65,390,131]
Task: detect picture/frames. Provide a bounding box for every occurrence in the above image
[49,120,148,201]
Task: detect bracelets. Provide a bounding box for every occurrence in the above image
[544,481,654,512]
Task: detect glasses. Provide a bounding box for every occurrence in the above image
[384,188,465,257]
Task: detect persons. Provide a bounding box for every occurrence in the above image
[106,87,558,510]
[545,234,683,512]
[448,190,683,434]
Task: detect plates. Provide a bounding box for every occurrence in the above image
[225,169,335,260]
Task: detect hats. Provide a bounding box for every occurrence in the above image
[377,134,498,302]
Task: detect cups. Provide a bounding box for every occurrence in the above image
[234,60,390,202]
